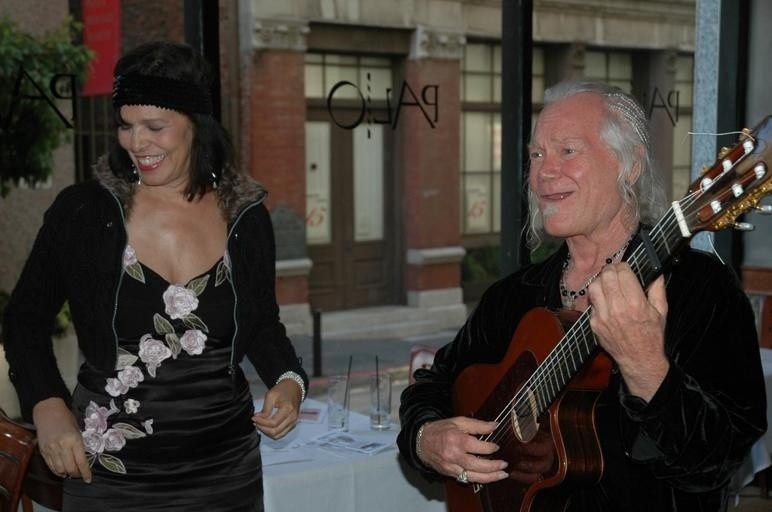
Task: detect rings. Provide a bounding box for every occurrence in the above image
[456,468,469,484]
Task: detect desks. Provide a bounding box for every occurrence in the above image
[252,393,451,510]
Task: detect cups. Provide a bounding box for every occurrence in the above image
[326,375,350,434]
[368,373,394,430]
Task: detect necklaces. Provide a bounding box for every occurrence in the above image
[559,231,634,312]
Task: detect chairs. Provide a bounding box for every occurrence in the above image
[0,411,40,512]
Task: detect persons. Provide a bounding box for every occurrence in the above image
[396,77,768,512]
[1,41,308,512]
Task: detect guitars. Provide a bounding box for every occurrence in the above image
[442,108,772,512]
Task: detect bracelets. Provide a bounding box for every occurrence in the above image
[415,421,429,467]
[276,370,306,402]
